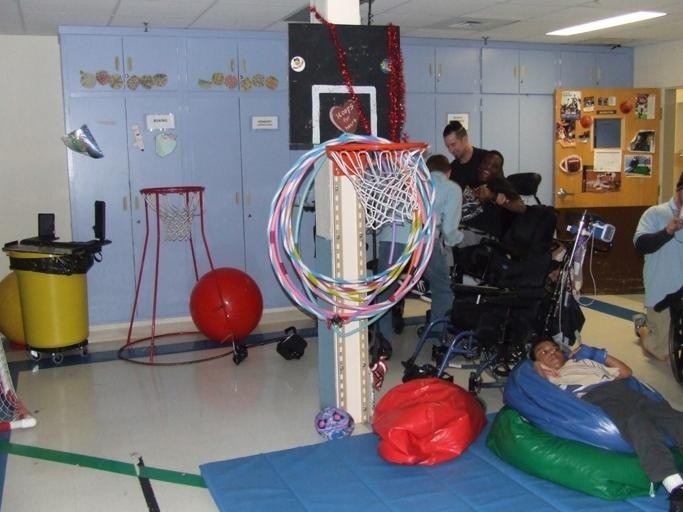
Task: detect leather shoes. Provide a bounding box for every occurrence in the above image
[432,346,455,361]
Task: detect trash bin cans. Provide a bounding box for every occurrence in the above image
[6,251,95,365]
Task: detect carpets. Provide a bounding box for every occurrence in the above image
[199,411,678,512]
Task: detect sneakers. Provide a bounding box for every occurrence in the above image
[671,484,683,511]
[633,314,648,336]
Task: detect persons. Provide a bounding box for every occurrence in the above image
[374,155,465,364]
[442,120,527,279]
[631,171,683,362]
[529,337,683,512]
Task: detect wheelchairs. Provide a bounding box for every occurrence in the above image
[664,296,681,387]
[401,202,615,417]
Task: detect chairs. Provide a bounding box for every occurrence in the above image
[451,173,559,342]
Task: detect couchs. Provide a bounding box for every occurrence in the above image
[370,345,676,502]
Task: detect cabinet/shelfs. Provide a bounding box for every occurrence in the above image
[56,23,190,328]
[189,29,311,311]
[558,205,649,295]
[397,37,481,162]
[554,43,632,89]
[482,41,554,206]
